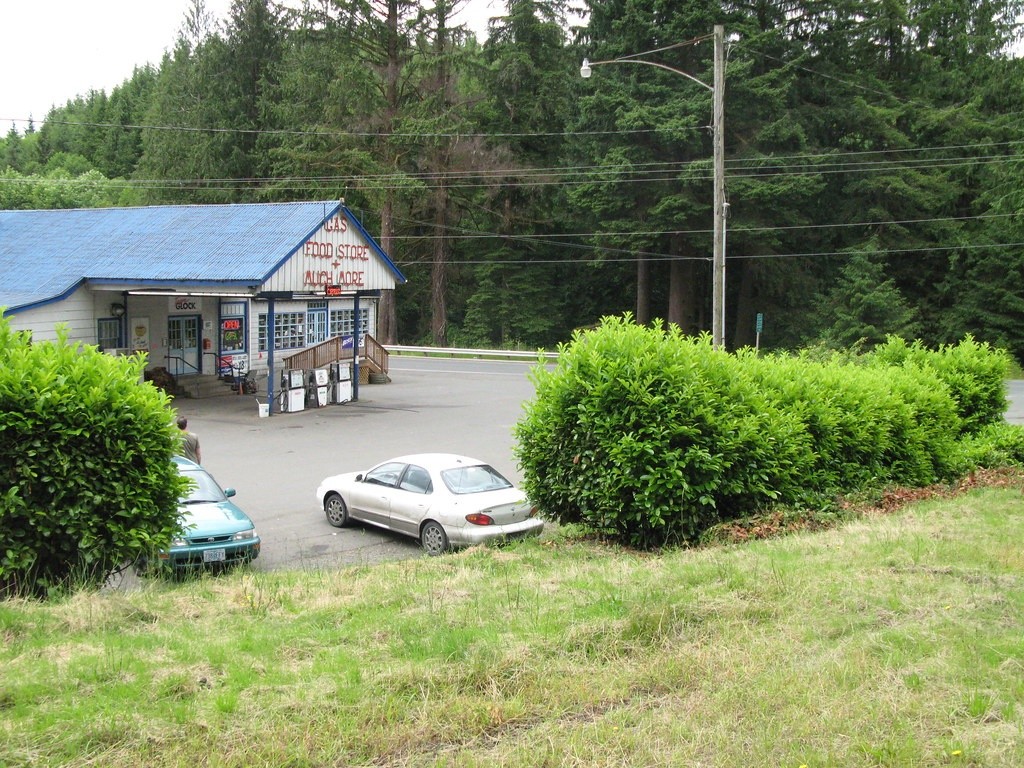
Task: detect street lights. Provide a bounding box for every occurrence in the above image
[580,24,741,352]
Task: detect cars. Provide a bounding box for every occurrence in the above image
[317,452,544,559]
[131,454,262,581]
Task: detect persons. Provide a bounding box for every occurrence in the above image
[168,415,202,466]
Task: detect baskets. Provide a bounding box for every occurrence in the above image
[359,366,369,385]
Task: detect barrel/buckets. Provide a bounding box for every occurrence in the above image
[259,404,269,417]
